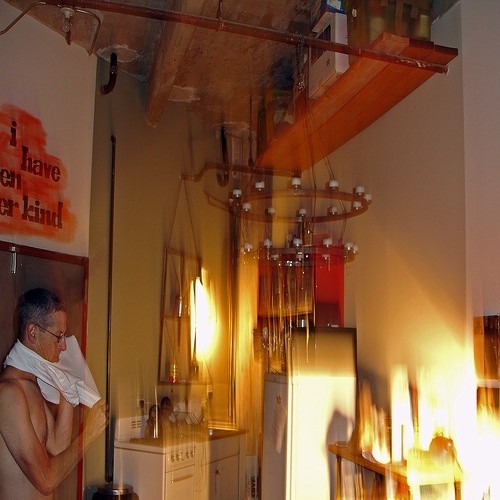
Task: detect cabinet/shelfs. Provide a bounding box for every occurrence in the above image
[158,314,210,386]
[114,435,249,500]
[256,244,344,332]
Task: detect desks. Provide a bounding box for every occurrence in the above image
[328,444,463,500]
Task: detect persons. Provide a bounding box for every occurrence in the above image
[0,289,109,500]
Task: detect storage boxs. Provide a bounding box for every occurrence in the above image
[259,0,432,151]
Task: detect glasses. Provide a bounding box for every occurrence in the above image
[35,323,71,344]
